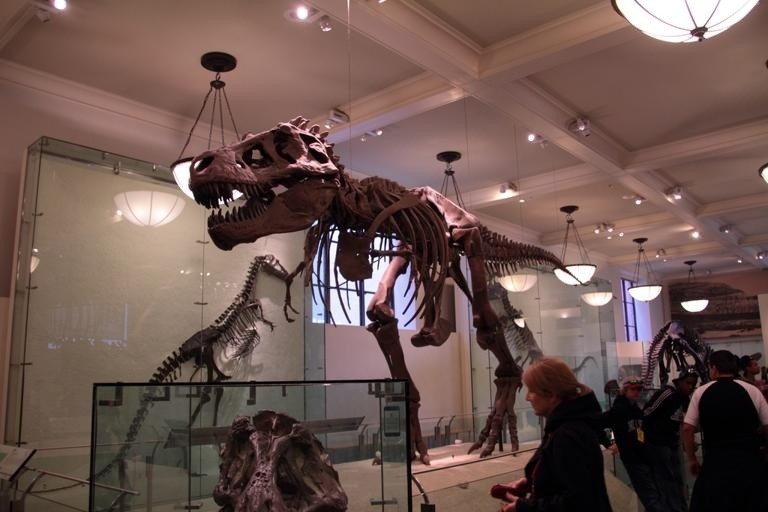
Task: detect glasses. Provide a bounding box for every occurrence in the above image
[623,379,644,384]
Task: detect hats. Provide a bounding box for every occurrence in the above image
[672,370,698,389]
[622,376,642,388]
[739,353,762,368]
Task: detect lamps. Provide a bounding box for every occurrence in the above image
[754,251,765,261]
[593,224,601,235]
[293,4,318,21]
[568,113,592,137]
[678,259,709,314]
[579,271,616,307]
[610,1,759,44]
[527,132,548,148]
[635,196,642,204]
[112,191,186,229]
[664,183,685,201]
[360,134,369,143]
[553,204,596,287]
[499,179,521,195]
[324,109,350,130]
[628,235,663,302]
[169,51,245,207]
[656,249,661,258]
[319,15,332,33]
[720,222,735,233]
[497,197,537,292]
[736,257,743,264]
[605,221,614,233]
[375,127,383,137]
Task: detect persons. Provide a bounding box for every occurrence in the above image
[740,352,762,381]
[755,366,768,385]
[597,376,674,512]
[641,370,699,512]
[681,349,768,512]
[501,356,613,512]
